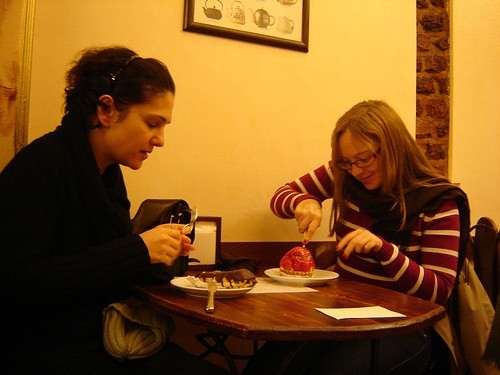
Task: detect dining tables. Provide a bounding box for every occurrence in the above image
[138,264,447,374]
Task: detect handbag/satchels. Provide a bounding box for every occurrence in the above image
[458,235,500,375]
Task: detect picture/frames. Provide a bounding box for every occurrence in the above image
[183,0,310,53]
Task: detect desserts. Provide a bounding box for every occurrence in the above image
[187,269,258,289]
[279,246,316,278]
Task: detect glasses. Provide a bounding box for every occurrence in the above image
[167,207,198,236]
[335,146,382,170]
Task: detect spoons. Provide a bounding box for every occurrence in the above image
[205,281,217,313]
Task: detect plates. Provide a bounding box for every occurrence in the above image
[264,267,339,287]
[170,276,252,298]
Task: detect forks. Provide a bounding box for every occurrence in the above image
[182,208,197,230]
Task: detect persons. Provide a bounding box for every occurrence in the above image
[0,44,198,375]
[268,99,461,374]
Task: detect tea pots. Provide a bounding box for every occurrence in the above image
[203,0,295,34]
